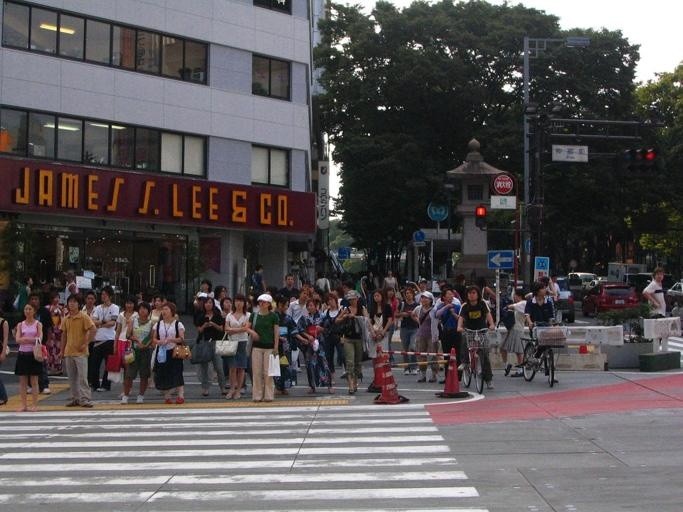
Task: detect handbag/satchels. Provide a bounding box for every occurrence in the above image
[104,348,135,384]
[33,344,48,362]
[500,306,515,330]
[214,340,238,357]
[279,355,289,368]
[332,317,356,338]
[173,345,192,361]
[266,353,281,377]
[190,341,216,364]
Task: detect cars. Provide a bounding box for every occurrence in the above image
[497,271,683,324]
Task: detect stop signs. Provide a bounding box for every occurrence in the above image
[493,174,513,195]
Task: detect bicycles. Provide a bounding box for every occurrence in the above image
[461,327,491,395]
[518,321,568,387]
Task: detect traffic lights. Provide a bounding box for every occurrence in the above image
[620,146,658,163]
[474,206,487,229]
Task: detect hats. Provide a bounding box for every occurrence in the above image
[420,290,434,300]
[346,289,358,301]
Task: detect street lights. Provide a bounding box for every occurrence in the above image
[520,101,562,294]
[442,183,457,253]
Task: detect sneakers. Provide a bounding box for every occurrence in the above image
[175,396,185,404]
[66,400,80,407]
[201,383,290,403]
[503,363,512,377]
[14,406,39,412]
[120,395,129,405]
[81,402,94,408]
[135,395,145,404]
[429,377,437,382]
[42,388,51,395]
[308,385,358,395]
[164,398,172,404]
[511,371,525,378]
[485,378,495,389]
[26,387,33,394]
[416,377,426,383]
[96,387,108,392]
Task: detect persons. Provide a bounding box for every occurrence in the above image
[1,262,579,409]
[641,266,667,343]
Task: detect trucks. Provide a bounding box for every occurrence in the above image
[604,261,649,287]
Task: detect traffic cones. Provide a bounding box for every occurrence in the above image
[365,344,392,393]
[371,360,408,406]
[437,347,469,398]
